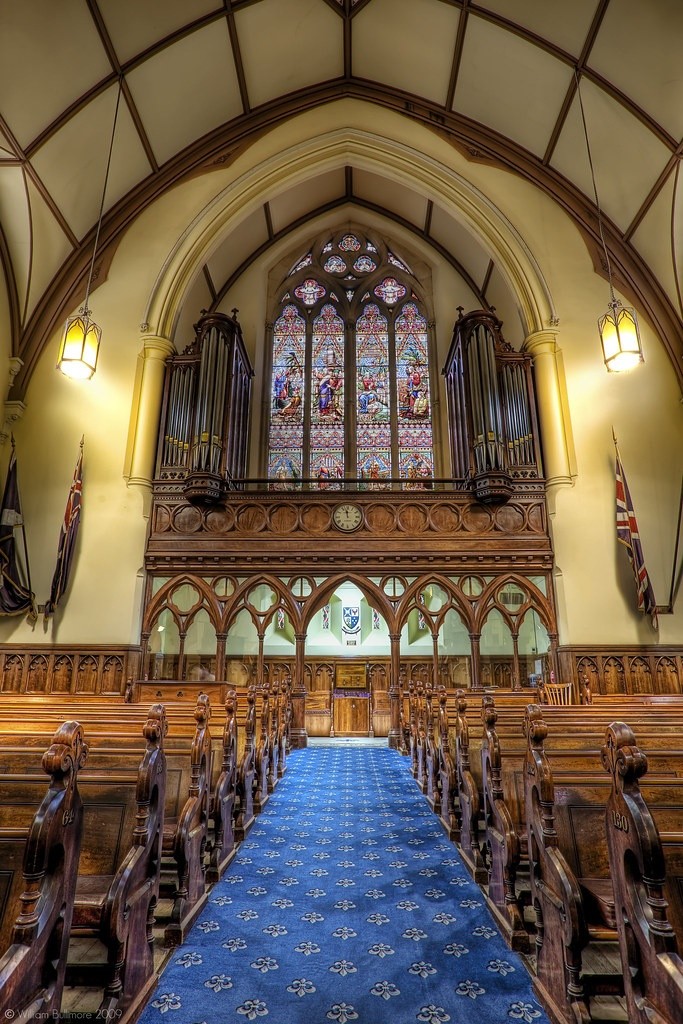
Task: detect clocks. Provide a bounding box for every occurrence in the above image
[334,502,363,532]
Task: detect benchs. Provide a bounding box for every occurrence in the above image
[399,676,683,1024]
[0,679,292,1024]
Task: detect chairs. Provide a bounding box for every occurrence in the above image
[542,682,572,705]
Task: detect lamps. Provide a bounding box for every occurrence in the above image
[573,61,643,376]
[58,73,122,380]
